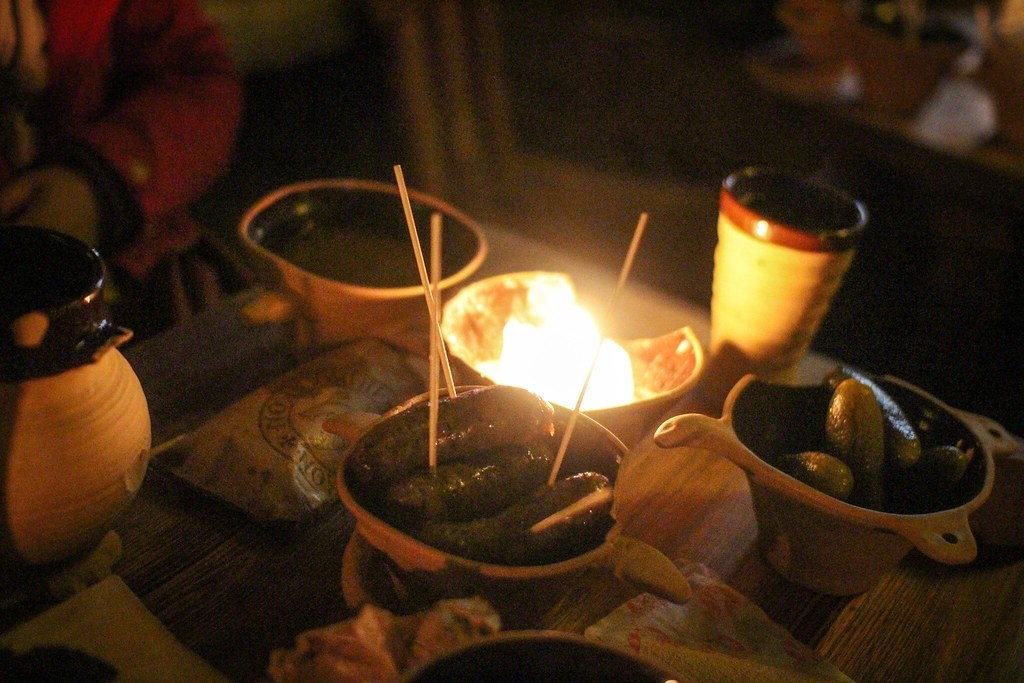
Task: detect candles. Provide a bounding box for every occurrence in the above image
[440,267,703,433]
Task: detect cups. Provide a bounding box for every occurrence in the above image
[705,166,867,405]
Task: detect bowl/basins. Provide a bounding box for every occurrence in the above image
[337,385,628,589]
[441,270,704,449]
[653,366,1019,598]
[237,177,489,363]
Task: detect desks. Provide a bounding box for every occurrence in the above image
[104,226,1024,683]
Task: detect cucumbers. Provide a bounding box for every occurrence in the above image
[750,365,978,518]
[345,383,614,565]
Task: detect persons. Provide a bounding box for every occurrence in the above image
[1,1,241,344]
